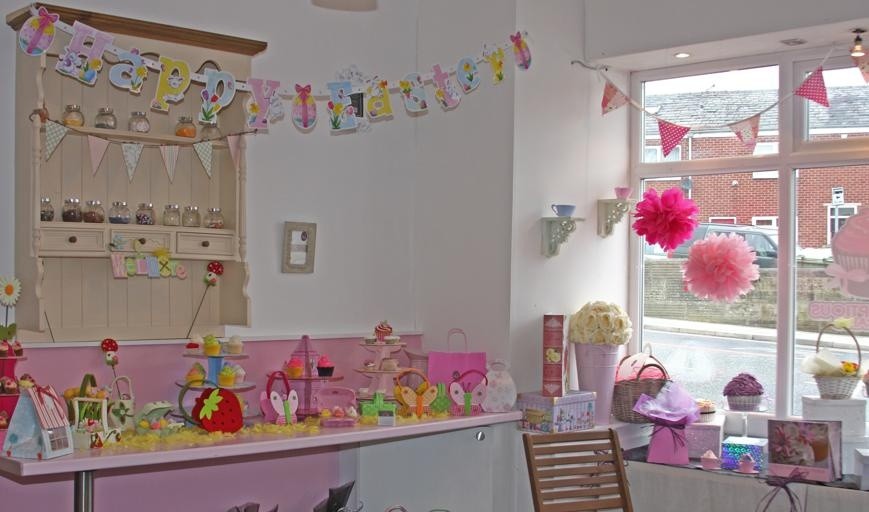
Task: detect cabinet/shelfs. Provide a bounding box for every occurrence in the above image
[4,2,252,343]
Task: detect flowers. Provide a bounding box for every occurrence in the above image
[840,360,858,378]
[722,371,766,398]
[567,298,634,346]
[0,277,23,341]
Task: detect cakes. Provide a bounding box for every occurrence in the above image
[281,353,334,377]
[701,449,718,469]
[184,331,246,386]
[738,453,756,472]
[361,319,402,371]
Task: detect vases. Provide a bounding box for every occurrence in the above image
[573,342,620,425]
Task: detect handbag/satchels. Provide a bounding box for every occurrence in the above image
[6,385,74,462]
[767,418,845,486]
[428,331,488,412]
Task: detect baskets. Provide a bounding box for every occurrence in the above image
[812,374,862,400]
[612,378,675,425]
[727,396,764,412]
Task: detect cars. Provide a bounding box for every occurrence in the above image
[667,222,832,268]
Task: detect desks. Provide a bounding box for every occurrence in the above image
[2,404,525,512]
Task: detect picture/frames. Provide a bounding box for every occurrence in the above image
[282,220,317,275]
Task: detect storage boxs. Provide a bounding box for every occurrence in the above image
[517,390,598,434]
[801,395,869,490]
[683,410,775,472]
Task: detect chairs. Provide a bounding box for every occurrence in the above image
[522,426,634,512]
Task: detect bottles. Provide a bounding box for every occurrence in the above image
[41,197,225,229]
[59,104,196,142]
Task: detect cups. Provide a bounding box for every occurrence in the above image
[550,204,576,217]
[615,187,633,200]
[701,457,719,468]
[738,460,755,474]
